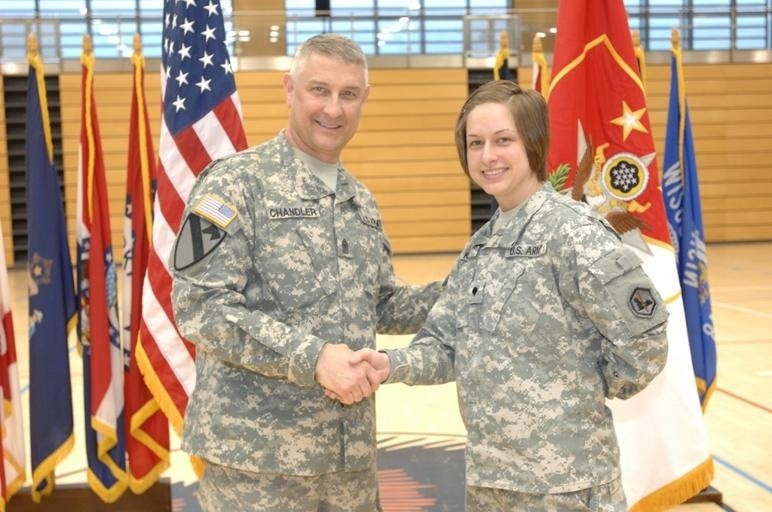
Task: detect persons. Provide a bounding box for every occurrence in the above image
[322,80,669,512]
[172,34,447,511]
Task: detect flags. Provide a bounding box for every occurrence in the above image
[197,196,235,224]
[2,2,246,504]
[493,0,717,512]
